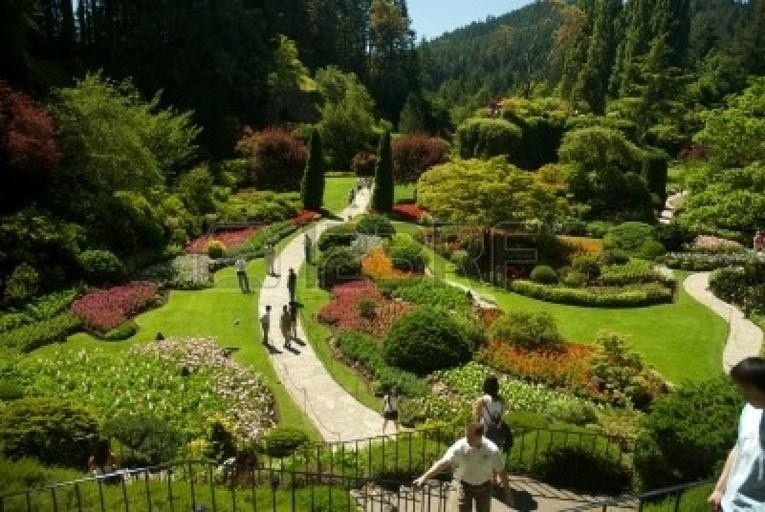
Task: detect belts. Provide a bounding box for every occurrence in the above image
[460,479,490,490]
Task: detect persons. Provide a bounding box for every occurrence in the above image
[413,421,515,511]
[366,179,371,195]
[381,382,401,433]
[707,357,765,511]
[288,300,304,339]
[260,304,272,345]
[234,254,252,294]
[347,188,358,208]
[278,304,292,348]
[474,376,514,497]
[287,266,297,302]
[302,231,313,263]
[262,239,277,276]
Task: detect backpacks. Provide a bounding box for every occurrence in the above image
[480,397,514,453]
